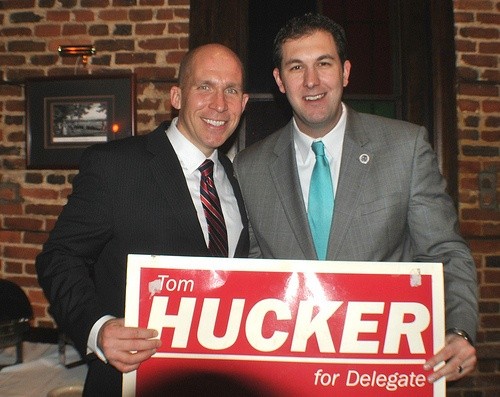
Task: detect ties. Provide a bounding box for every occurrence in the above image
[196,159,229,257]
[309,140,333,260]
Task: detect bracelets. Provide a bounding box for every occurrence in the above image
[446,329,470,342]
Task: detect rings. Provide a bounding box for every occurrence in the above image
[457,366,463,373]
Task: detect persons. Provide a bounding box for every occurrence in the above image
[232,12,480,384]
[34,44,249,397]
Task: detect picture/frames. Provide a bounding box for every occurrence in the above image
[24,73,137,170]
